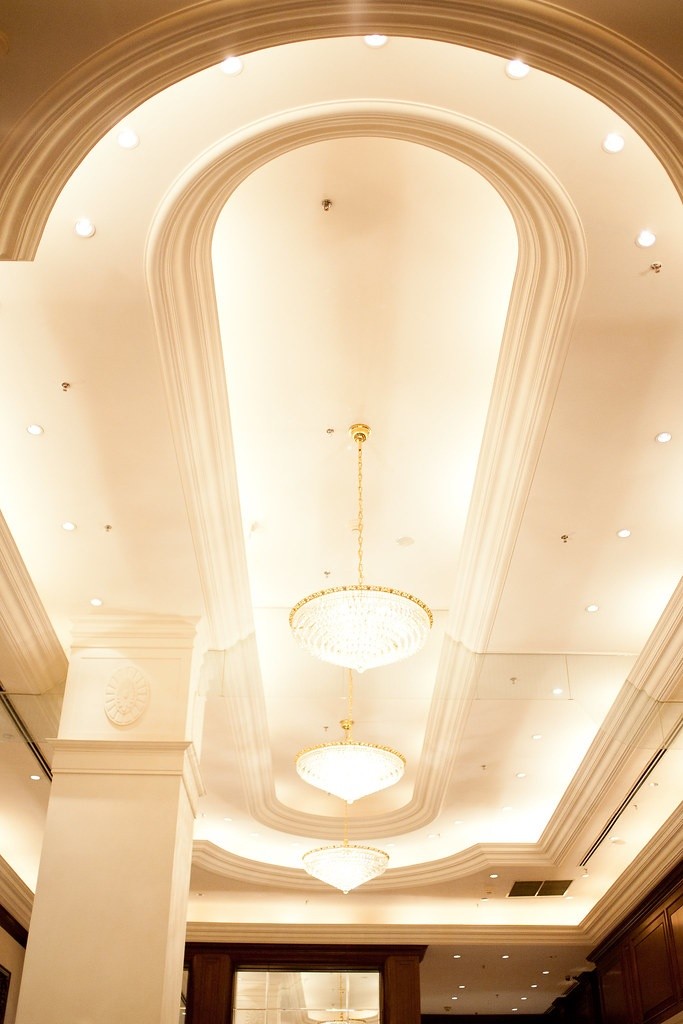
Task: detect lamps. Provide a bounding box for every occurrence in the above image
[300,801,390,894]
[293,668,407,805]
[320,973,366,1024]
[287,422,434,675]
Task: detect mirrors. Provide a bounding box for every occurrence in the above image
[231,969,383,1024]
